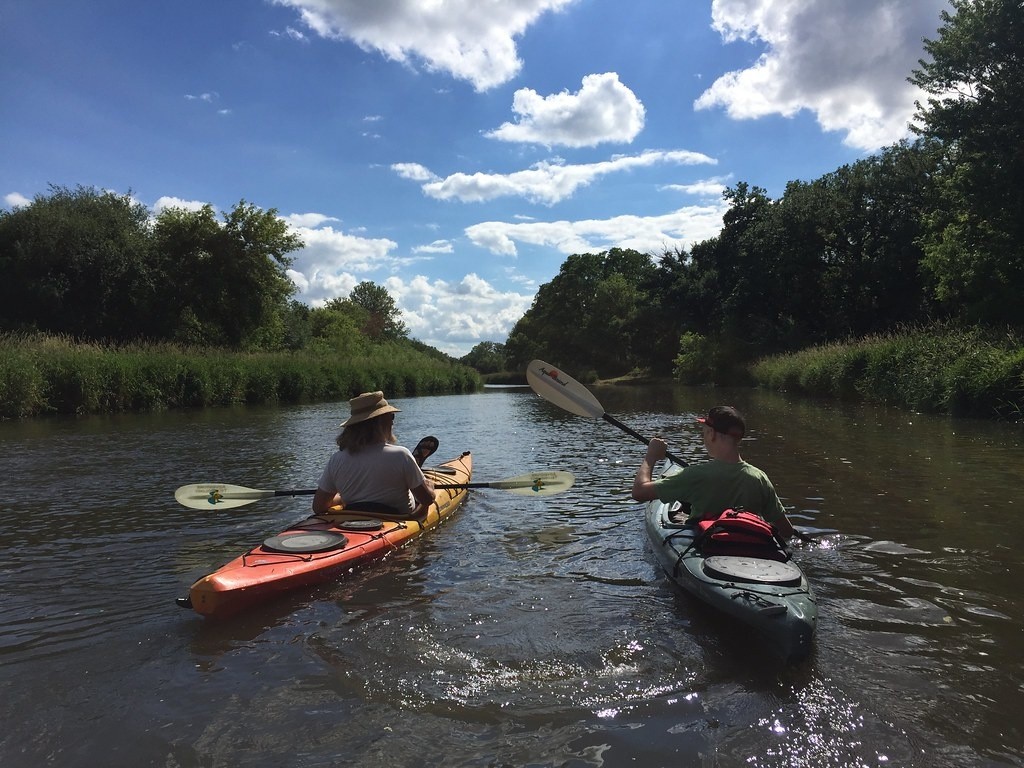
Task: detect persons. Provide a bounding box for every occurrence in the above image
[312,390,435,515]
[632,404,794,545]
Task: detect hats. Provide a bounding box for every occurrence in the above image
[339,391,402,427]
[695,406,744,437]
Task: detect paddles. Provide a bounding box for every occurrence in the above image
[171,470,575,511]
[523,356,815,541]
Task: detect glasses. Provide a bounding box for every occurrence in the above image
[387,413,394,420]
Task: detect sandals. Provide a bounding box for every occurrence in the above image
[411,436,439,468]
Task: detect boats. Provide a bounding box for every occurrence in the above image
[173,450,473,617]
[644,458,820,647]
